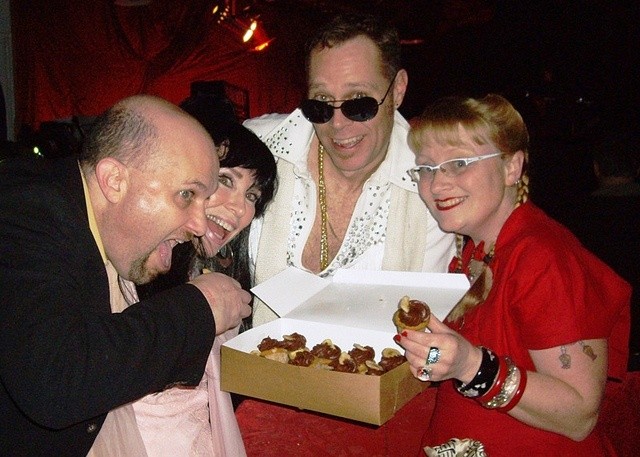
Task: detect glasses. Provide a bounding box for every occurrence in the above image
[300,80,393,123]
[407,150,505,181]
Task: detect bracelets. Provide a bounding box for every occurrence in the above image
[480,361,521,408]
[475,355,507,402]
[497,365,528,413]
[454,345,500,398]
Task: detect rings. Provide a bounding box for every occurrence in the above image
[425,346,441,365]
[416,365,433,381]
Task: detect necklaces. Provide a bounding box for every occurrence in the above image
[318,141,328,272]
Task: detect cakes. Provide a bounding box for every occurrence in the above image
[250,332,409,390]
[392,295,431,339]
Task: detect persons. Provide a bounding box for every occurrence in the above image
[242,13,458,328]
[1,93,252,457]
[393,91,640,457]
[146,119,277,335]
